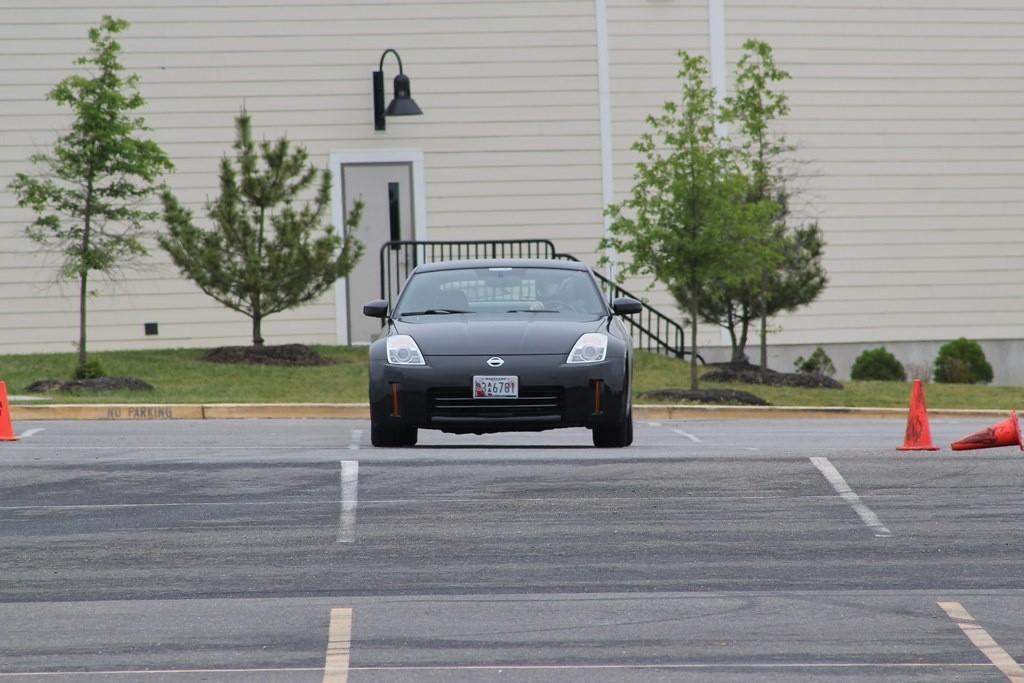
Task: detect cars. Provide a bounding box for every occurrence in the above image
[363,259,642,448]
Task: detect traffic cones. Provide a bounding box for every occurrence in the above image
[896,378,941,451]
[0,380,21,441]
[950,409,1024,452]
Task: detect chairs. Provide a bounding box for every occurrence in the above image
[431,290,472,314]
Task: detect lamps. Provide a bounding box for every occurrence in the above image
[372,47,424,130]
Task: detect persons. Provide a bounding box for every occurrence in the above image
[530,273,588,313]
